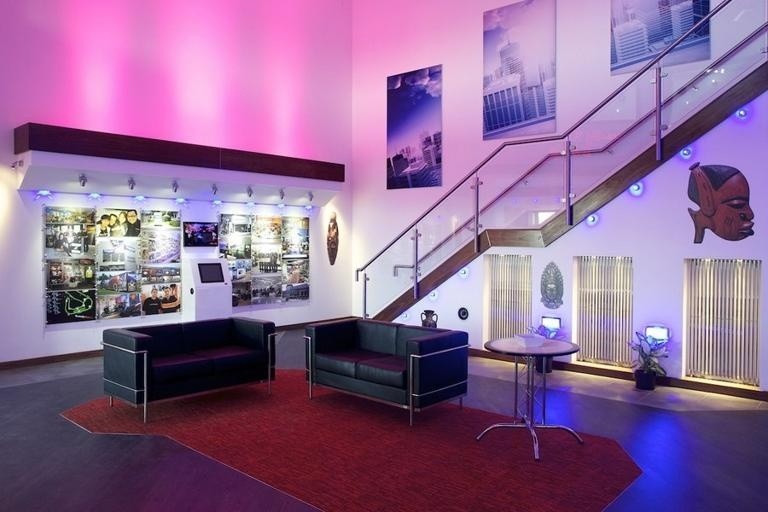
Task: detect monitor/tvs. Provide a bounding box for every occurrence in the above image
[197,262,225,283]
[183,221,218,248]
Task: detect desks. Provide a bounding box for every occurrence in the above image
[476,337,584,460]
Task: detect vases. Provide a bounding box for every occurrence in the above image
[420,309,438,329]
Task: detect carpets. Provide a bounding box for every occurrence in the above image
[60,367,646,512]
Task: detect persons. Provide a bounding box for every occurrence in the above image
[44,207,181,321]
[184,221,216,244]
[216,217,308,304]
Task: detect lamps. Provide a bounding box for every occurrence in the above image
[30,170,313,213]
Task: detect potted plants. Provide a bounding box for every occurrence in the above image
[525,324,562,374]
[628,331,668,391]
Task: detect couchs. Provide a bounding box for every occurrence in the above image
[302,317,470,426]
[101,316,277,428]
